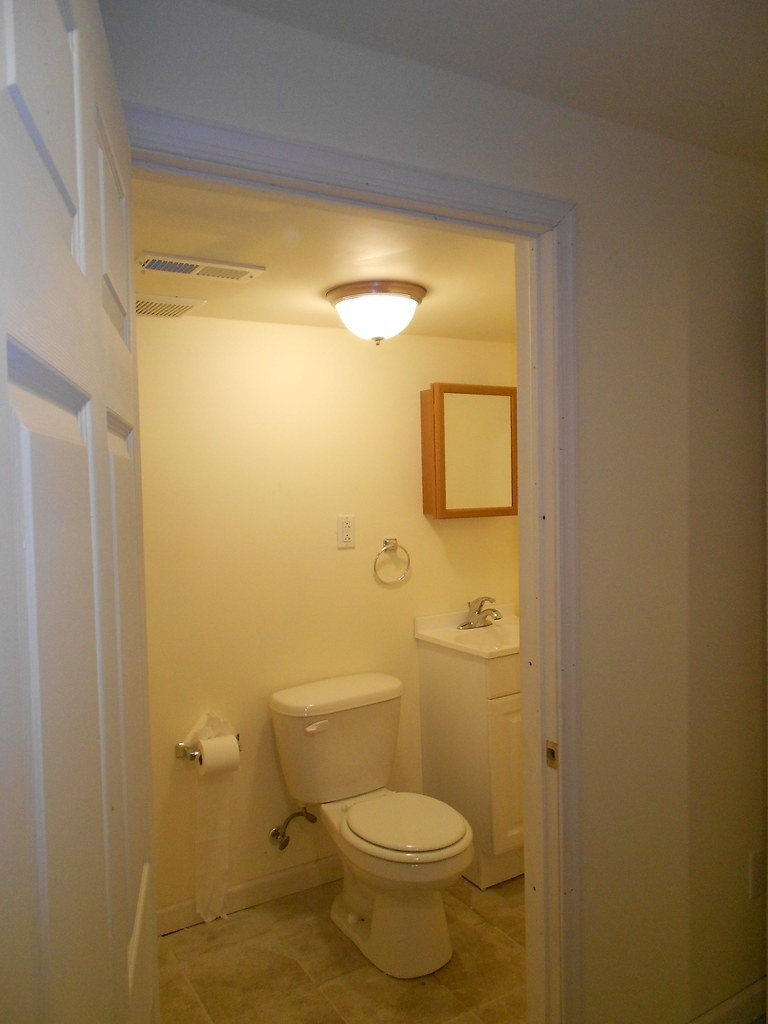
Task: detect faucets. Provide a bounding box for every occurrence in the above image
[457,596,503,629]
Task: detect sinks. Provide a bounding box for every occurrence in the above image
[453,621,519,658]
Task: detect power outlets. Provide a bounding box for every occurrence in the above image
[336,515,356,549]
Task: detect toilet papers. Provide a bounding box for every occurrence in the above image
[183,734,241,777]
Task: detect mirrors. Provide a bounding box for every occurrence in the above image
[443,392,514,510]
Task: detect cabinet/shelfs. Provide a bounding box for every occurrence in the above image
[420,382,519,520]
[417,639,526,891]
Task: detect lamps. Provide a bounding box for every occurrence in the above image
[326,280,428,345]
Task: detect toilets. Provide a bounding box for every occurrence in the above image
[269,670,477,980]
